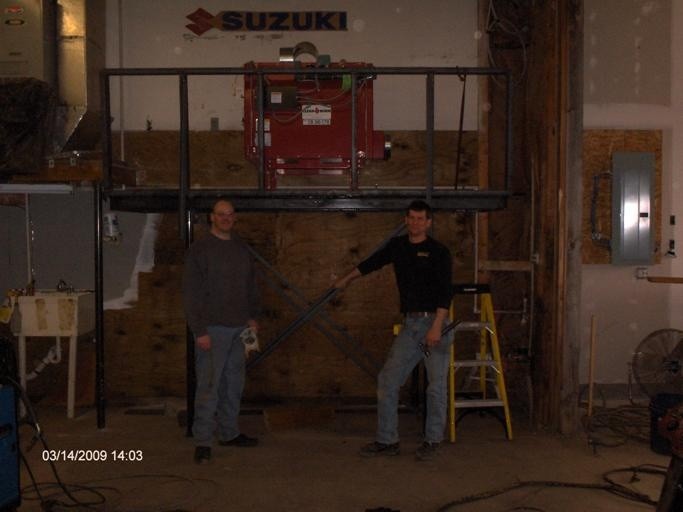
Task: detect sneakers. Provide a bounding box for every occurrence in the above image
[223,435,260,447]
[413,442,445,458]
[360,441,401,457]
[195,445,212,464]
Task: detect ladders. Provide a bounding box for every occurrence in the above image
[449,284,512,444]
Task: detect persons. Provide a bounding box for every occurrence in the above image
[179,199,261,465]
[331,199,456,461]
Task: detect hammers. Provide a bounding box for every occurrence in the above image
[419,320,463,358]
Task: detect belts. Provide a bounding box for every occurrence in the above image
[406,311,438,319]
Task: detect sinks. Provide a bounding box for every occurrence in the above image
[18,292,98,338]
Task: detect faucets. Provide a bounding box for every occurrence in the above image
[58,278,67,292]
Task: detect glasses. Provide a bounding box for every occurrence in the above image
[213,212,237,217]
